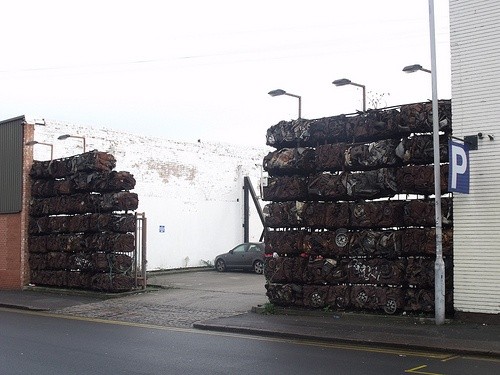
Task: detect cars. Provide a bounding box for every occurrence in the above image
[215,241,265,275]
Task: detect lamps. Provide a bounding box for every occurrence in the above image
[26,141,53,160]
[332,78,366,111]
[58,134,86,154]
[268,90,302,119]
[402,64,431,75]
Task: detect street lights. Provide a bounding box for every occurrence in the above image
[56,134,87,154]
[25,140,53,161]
[270,88,302,118]
[334,78,366,112]
[402,63,432,77]
